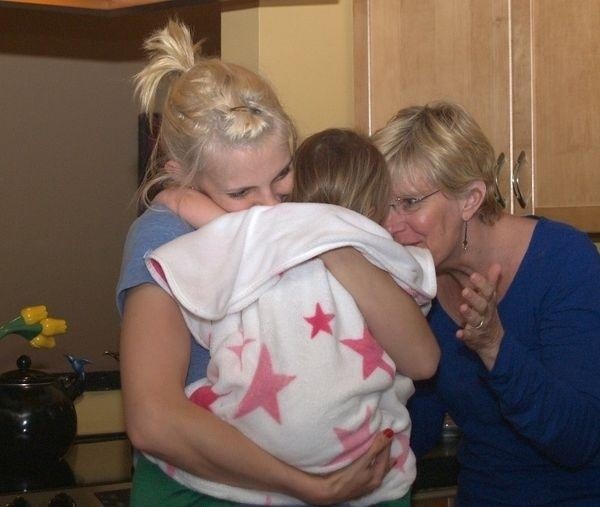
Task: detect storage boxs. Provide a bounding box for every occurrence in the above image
[67,438,133,483]
[54,370,127,437]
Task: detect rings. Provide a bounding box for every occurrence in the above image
[476,320,484,330]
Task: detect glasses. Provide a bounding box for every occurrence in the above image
[386,187,445,214]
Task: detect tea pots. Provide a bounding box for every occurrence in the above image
[0,356,87,466]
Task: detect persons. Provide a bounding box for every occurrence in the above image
[116,20,443,506]
[152,126,390,230]
[369,100,600,507]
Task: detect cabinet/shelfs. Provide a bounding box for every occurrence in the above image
[351,0,600,236]
